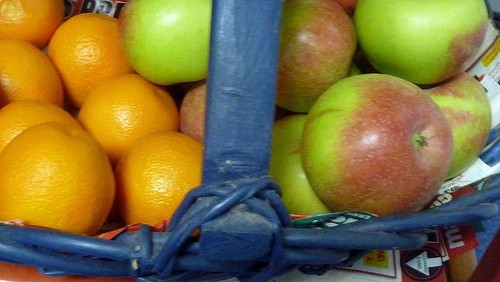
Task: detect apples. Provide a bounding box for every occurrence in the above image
[118,0,492,224]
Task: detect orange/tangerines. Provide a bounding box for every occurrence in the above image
[0,0,203,234]
